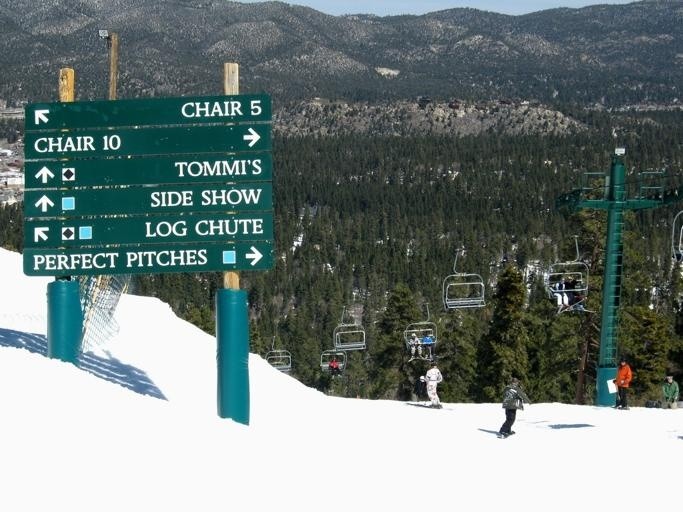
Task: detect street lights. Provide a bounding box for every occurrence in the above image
[98,28,120,100]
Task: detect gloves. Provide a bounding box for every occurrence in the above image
[672,398,674,403]
[666,397,669,401]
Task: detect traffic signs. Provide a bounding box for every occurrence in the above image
[24,93,271,125]
[19,243,278,271]
[22,123,273,157]
[22,212,275,239]
[22,155,273,184]
[22,182,273,216]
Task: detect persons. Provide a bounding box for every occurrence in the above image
[423,362,443,404]
[407,332,423,363]
[663,373,679,409]
[329,356,341,380]
[421,330,434,359]
[497,377,532,438]
[413,379,423,401]
[554,277,568,307]
[565,276,580,306]
[614,358,632,409]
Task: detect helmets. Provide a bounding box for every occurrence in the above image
[431,360,438,366]
[411,333,416,337]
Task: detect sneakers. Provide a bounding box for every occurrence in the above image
[612,405,619,409]
[500,430,508,436]
[509,431,515,435]
[619,407,627,409]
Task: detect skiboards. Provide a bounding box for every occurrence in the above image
[498,432,515,439]
[417,404,441,409]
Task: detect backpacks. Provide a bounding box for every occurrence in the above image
[645,400,662,408]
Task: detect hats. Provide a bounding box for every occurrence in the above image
[620,357,628,362]
[512,378,520,382]
